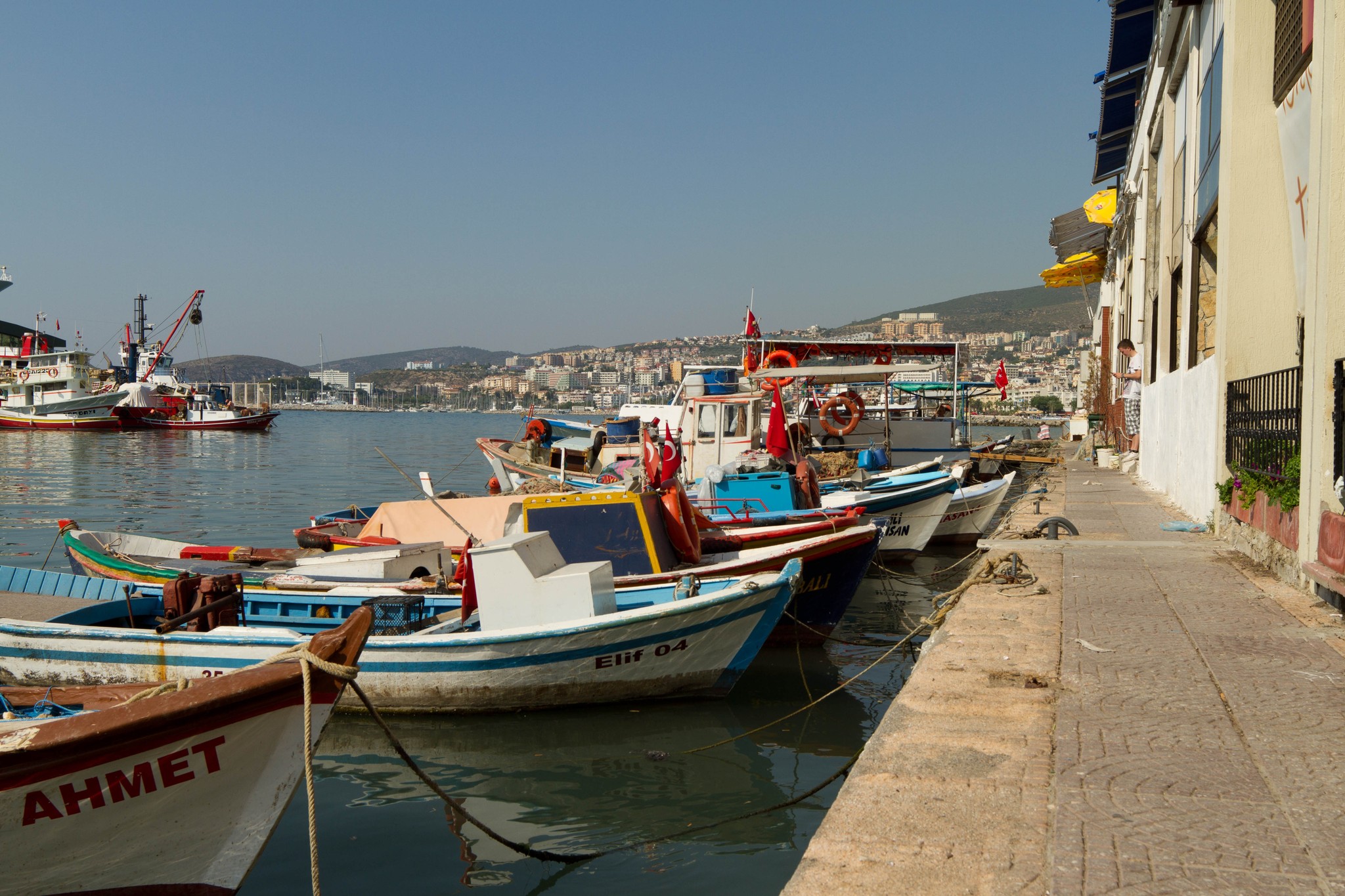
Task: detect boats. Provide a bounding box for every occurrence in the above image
[1,286,1018,896]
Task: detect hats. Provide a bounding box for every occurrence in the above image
[1041,421,1045,424]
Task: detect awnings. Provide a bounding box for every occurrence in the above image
[1049,207,1108,264]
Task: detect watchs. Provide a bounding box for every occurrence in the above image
[1121,373,1124,378]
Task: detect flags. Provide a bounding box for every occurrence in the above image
[56,318,60,331]
[454,535,478,625]
[643,427,661,489]
[994,360,1008,401]
[661,423,682,484]
[191,383,195,393]
[745,307,762,339]
[747,343,757,373]
[767,384,789,458]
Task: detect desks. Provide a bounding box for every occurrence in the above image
[549,435,595,474]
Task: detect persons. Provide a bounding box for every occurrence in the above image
[923,406,961,436]
[1111,339,1142,463]
[1037,421,1051,440]
[890,412,902,421]
[992,415,996,421]
[225,399,234,410]
[1135,100,1140,107]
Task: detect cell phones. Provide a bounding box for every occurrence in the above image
[1108,371,1112,373]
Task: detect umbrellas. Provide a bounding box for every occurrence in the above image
[1083,188,1117,228]
[1038,252,1107,325]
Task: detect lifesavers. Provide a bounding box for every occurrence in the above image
[936,404,958,417]
[659,479,703,564]
[762,350,798,387]
[830,390,865,425]
[819,397,858,436]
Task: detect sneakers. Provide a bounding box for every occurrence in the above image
[1119,448,1132,458]
[1121,452,1139,462]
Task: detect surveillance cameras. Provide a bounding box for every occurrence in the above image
[1078,323,1085,329]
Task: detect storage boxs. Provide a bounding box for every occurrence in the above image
[361,595,425,636]
